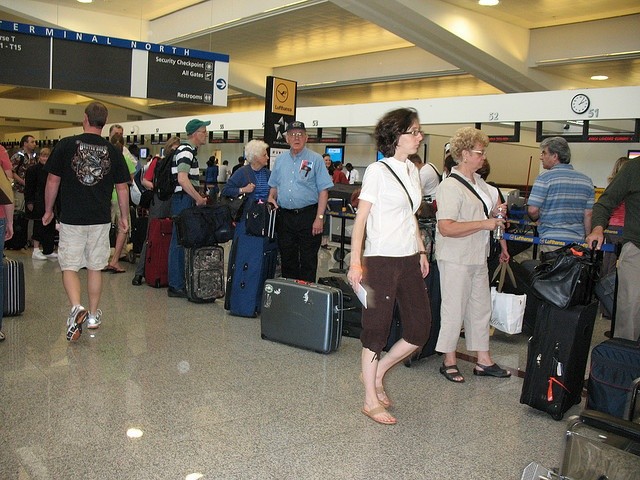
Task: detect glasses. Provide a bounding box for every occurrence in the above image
[402,130,421,136]
[470,150,485,156]
[287,132,306,138]
[196,130,206,134]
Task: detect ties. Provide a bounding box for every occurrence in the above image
[348,172,350,182]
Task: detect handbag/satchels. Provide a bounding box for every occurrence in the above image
[447,172,500,271]
[245,198,274,238]
[530,243,599,309]
[173,204,236,248]
[489,260,526,335]
[219,166,251,223]
[583,336,640,425]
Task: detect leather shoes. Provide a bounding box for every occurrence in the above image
[167,286,187,299]
[132,275,142,286]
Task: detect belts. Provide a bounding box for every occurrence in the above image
[279,203,318,215]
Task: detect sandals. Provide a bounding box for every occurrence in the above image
[439,361,465,383]
[473,363,512,378]
[359,372,390,408]
[361,400,397,425]
[107,262,126,273]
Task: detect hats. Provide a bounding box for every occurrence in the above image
[286,121,306,132]
[185,119,211,135]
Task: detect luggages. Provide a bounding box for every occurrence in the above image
[224,207,276,318]
[382,228,444,367]
[0,252,25,316]
[133,216,148,253]
[5,199,27,251]
[317,275,362,340]
[561,378,640,480]
[519,240,601,421]
[260,277,356,354]
[144,216,173,289]
[182,194,225,304]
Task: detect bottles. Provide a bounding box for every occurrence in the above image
[493,205,504,240]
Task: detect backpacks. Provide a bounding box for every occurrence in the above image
[130,153,161,207]
[154,143,195,201]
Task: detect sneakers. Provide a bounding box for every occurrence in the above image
[45,252,58,258]
[31,249,47,260]
[67,304,89,341]
[86,309,102,329]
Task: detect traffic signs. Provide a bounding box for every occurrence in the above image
[264,76,298,168]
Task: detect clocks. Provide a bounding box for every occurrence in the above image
[570,94,590,114]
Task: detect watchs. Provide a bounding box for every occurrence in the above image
[316,215,323,219]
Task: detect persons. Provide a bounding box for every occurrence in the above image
[132,137,181,286]
[0,166,14,241]
[442,142,451,180]
[230,156,246,176]
[167,119,208,299]
[24,148,58,260]
[268,121,334,284]
[0,145,18,258]
[205,156,220,201]
[477,158,510,264]
[332,161,348,184]
[323,153,334,175]
[585,155,640,341]
[434,128,512,383]
[345,163,359,184]
[11,135,36,249]
[347,107,430,424]
[408,154,441,204]
[217,160,232,193]
[42,102,132,342]
[444,155,458,176]
[109,124,138,167]
[527,137,595,254]
[222,139,271,243]
[108,133,136,274]
[602,157,630,321]
